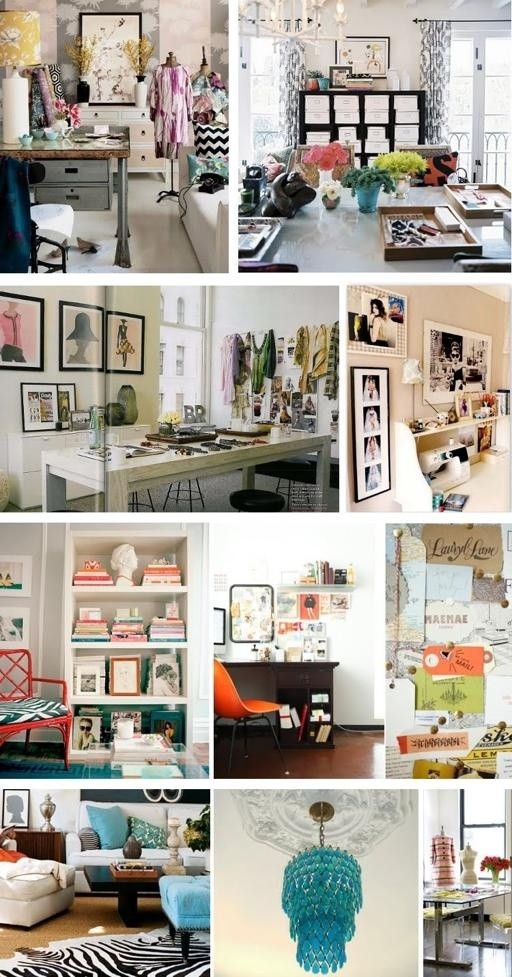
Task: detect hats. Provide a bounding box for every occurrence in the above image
[120,318,128,322]
[64,312,101,342]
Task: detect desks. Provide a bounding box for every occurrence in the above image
[219,660,340,751]
[1,125,132,270]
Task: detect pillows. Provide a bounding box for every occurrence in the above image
[192,121,229,158]
[128,816,167,849]
[86,805,130,849]
[187,154,229,184]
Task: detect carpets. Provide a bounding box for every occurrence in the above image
[0,925,210,977]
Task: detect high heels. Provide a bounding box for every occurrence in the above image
[75,235,102,256]
[49,237,72,262]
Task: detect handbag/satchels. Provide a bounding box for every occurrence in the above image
[456,166,470,183]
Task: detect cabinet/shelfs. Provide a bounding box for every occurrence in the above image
[13,828,64,865]
[7,423,152,510]
[68,104,168,183]
[394,408,510,512]
[298,89,426,170]
[70,529,188,764]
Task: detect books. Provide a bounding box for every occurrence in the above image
[310,709,331,722]
[71,608,111,642]
[298,559,333,585]
[142,565,182,587]
[120,764,184,779]
[149,619,186,642]
[308,723,332,743]
[109,735,178,772]
[311,693,329,704]
[110,618,148,642]
[278,705,301,729]
[73,568,114,586]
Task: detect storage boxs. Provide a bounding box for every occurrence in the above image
[304,112,330,125]
[395,110,420,123]
[364,95,390,109]
[335,111,360,124]
[393,95,419,111]
[333,95,359,111]
[364,139,390,154]
[394,124,419,142]
[394,142,418,152]
[367,127,386,140]
[338,127,357,141]
[304,95,330,112]
[347,141,362,153]
[306,140,330,146]
[305,131,331,140]
[332,140,346,147]
[363,109,390,123]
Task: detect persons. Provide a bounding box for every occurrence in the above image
[430,836,457,884]
[110,544,138,586]
[115,319,136,367]
[0,300,28,363]
[154,664,179,697]
[447,342,466,391]
[367,299,389,346]
[76,718,99,750]
[190,63,229,122]
[148,51,194,160]
[458,845,478,884]
[304,594,317,620]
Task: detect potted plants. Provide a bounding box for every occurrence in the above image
[372,150,428,200]
[340,166,395,214]
[305,68,322,90]
[123,35,154,108]
[62,34,97,109]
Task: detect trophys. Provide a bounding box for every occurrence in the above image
[39,793,56,833]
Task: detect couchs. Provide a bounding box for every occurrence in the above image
[157,875,209,966]
[64,800,209,895]
[177,146,229,273]
[0,850,76,930]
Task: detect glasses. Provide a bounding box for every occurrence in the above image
[450,352,461,359]
[80,724,92,733]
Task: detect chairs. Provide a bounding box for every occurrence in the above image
[396,144,451,163]
[214,658,284,776]
[490,910,511,970]
[295,144,354,188]
[0,649,72,773]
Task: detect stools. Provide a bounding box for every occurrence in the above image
[276,458,312,511]
[162,477,202,511]
[423,906,463,941]
[128,487,156,512]
[229,489,285,512]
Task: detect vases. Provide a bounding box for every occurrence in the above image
[492,872,499,890]
[317,76,329,91]
[318,169,341,192]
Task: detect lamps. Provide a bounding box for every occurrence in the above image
[237,0,348,57]
[0,9,41,145]
[401,358,425,433]
[281,799,363,974]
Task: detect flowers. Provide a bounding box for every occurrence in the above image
[480,856,510,882]
[301,142,348,173]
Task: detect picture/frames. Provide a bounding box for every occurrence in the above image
[329,66,352,89]
[214,607,226,645]
[110,657,140,696]
[347,285,407,360]
[59,299,105,373]
[110,711,141,744]
[73,716,102,752]
[299,593,320,622]
[229,584,275,643]
[79,11,142,107]
[423,319,492,407]
[0,555,32,599]
[57,383,76,430]
[104,311,145,375]
[0,607,30,649]
[20,382,59,432]
[455,392,473,421]
[2,789,30,830]
[151,711,182,744]
[350,365,391,503]
[335,36,390,79]
[0,291,44,373]
